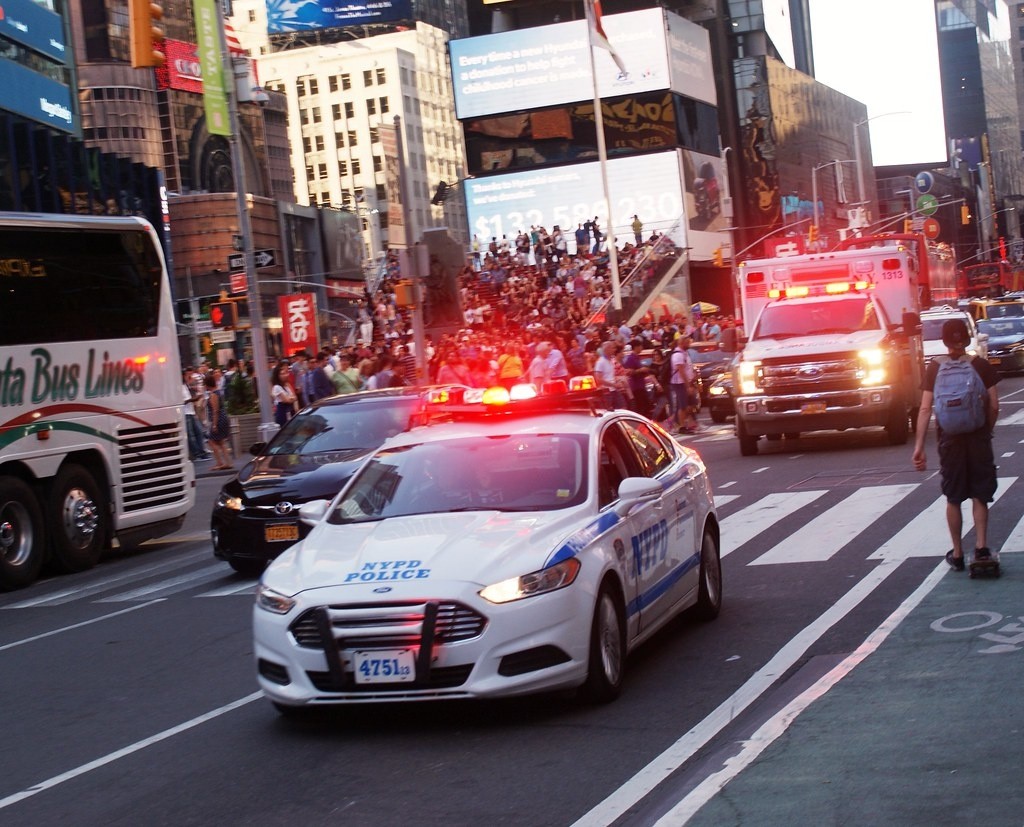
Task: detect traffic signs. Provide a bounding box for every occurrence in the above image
[227,247,278,270]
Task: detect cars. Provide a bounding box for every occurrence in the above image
[974,316,1024,375]
[211,386,483,576]
[251,378,726,715]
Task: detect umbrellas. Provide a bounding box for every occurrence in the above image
[688,300,721,315]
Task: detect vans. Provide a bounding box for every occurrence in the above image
[957,261,1024,320]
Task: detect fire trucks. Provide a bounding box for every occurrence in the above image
[732,246,934,450]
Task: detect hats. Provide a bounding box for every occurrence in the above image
[582,329,594,336]
[943,319,971,349]
[678,335,689,342]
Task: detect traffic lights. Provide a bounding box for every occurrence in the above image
[713,248,723,268]
[809,226,818,242]
[209,302,238,329]
[904,220,912,235]
[128,0,165,69]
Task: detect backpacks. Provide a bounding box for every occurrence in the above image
[661,351,686,385]
[932,354,988,437]
[638,334,654,349]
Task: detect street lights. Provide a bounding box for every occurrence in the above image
[973,203,1015,263]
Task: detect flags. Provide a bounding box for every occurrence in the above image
[586,0,628,80]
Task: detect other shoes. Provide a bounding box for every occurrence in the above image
[679,427,694,434]
[208,463,234,471]
[975,547,991,561]
[946,548,964,571]
[663,417,675,433]
[193,455,213,463]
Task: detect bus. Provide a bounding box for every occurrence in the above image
[0,210,196,587]
[837,235,958,311]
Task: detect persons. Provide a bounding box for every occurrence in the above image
[178,214,742,483]
[911,319,1002,570]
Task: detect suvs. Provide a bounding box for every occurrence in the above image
[921,308,989,387]
